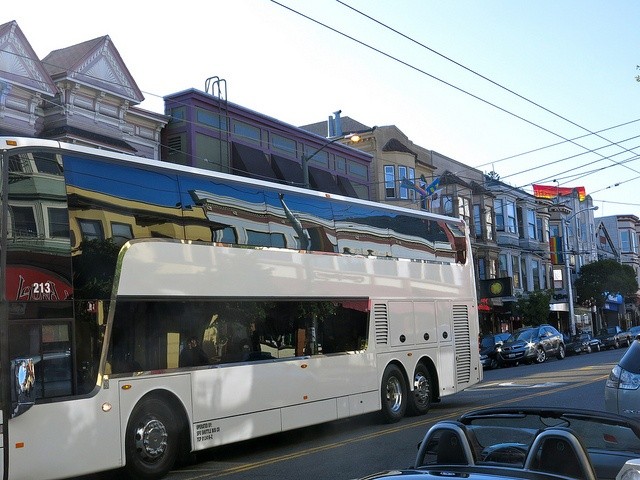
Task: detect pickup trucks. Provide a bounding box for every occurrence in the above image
[598,326,632,351]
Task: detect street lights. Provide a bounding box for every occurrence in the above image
[561,205,599,336]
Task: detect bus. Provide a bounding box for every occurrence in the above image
[0,136,484,480]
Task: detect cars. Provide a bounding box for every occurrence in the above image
[604,331,640,424]
[479,332,515,369]
[564,333,601,357]
[628,326,640,344]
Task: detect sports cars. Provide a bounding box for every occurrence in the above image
[354,405,639,479]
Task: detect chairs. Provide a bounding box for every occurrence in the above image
[413,420,478,467]
[523,425,599,480]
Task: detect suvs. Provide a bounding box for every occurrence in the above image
[493,323,566,368]
